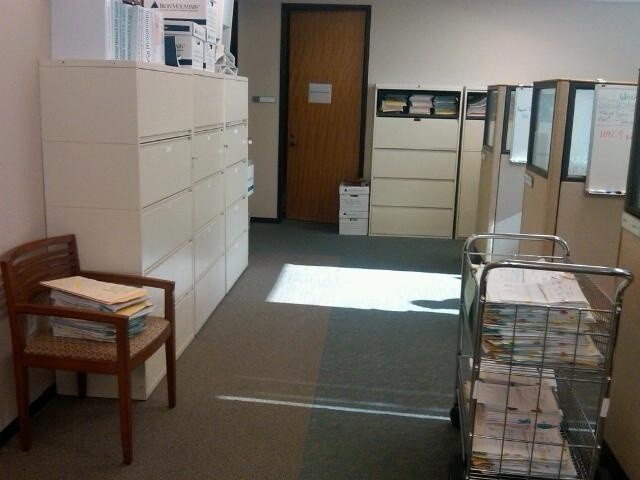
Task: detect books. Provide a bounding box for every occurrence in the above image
[40,275,155,342]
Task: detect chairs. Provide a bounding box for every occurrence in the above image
[1,234,178,466]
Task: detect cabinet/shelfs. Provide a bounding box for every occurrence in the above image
[447,231,636,476]
[367,82,464,240]
[454,87,488,241]
[35,58,250,403]
